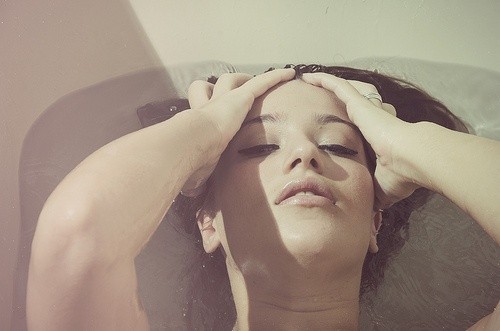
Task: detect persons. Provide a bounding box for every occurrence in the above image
[25,63,500,331]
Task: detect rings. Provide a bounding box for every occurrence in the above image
[363,91,384,104]
